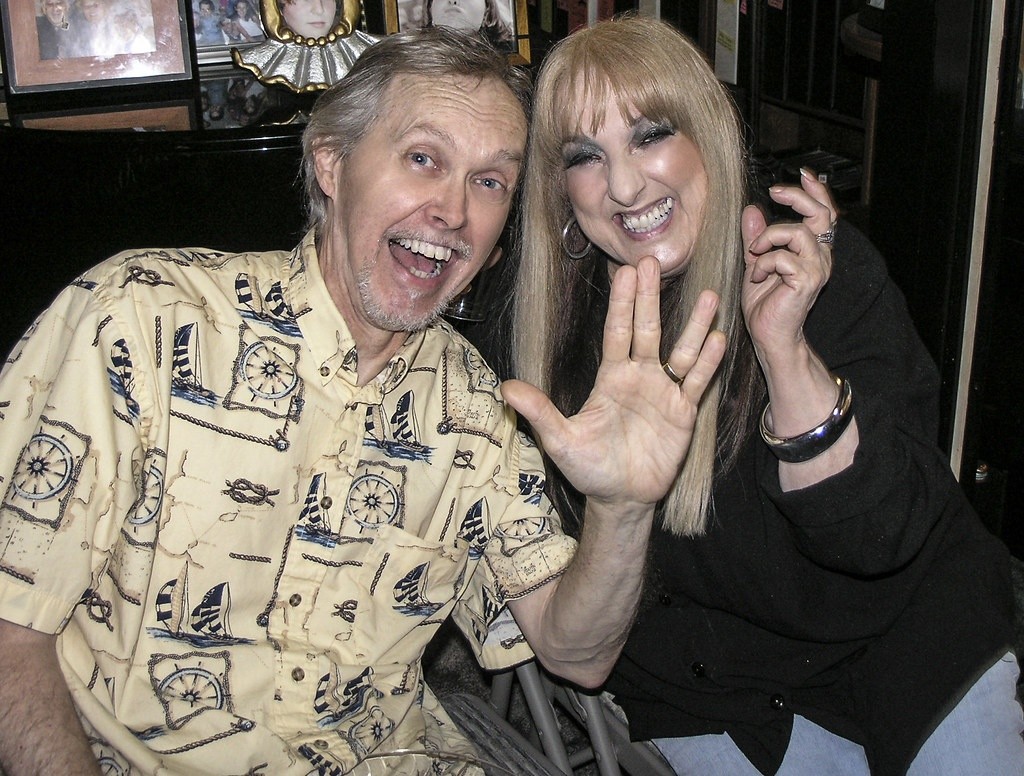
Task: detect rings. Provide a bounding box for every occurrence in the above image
[813,228,836,247]
[661,363,683,384]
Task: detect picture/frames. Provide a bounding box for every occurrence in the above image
[0,0,196,127]
[184,0,386,136]
[377,0,541,82]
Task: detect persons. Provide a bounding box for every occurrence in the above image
[466,16,1023,776]
[35,0,155,60]
[191,0,343,130]
[0,26,726,775]
[411,0,516,51]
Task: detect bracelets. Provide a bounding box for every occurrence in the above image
[758,377,854,465]
